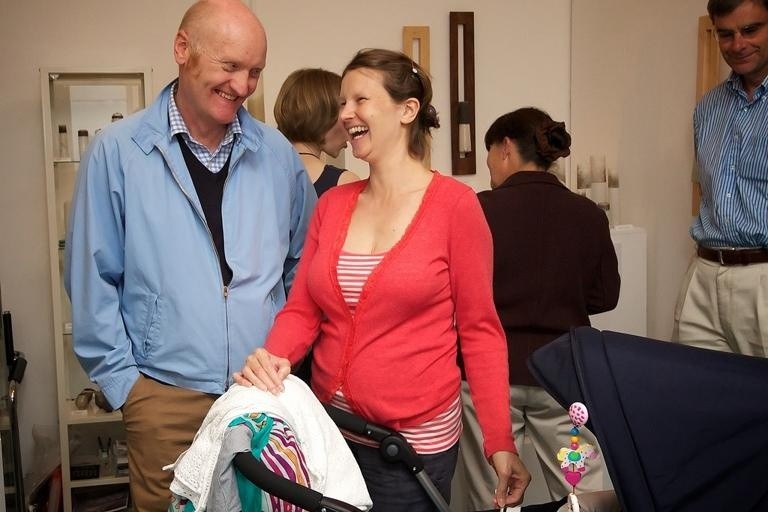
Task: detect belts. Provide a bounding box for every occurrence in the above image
[697,244,768,267]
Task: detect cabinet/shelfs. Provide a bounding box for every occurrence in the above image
[39,64,154,512]
[0,378,27,512]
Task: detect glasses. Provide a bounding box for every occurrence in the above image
[712,21,768,42]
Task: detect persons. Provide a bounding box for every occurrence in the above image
[454,108,624,510]
[59,0,318,512]
[267,67,364,386]
[675,1,768,358]
[231,44,533,512]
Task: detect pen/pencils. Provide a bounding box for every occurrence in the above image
[98,434,112,456]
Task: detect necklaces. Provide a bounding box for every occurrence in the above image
[296,151,322,160]
